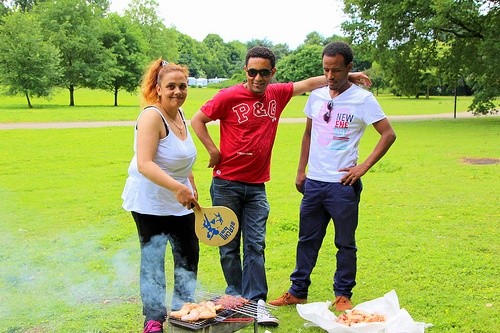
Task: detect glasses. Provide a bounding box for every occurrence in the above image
[245,67,273,76]
[323,100,333,124]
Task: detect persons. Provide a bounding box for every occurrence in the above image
[121,56,201,333]
[190,45,372,327]
[267,40,396,311]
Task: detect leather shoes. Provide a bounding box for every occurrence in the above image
[269,291,307,305]
[331,294,352,310]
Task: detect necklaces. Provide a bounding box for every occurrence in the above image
[170,110,184,135]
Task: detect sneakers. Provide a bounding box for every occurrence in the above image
[143,319,163,333]
[256,299,279,326]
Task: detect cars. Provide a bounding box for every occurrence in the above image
[187,76,228,88]
